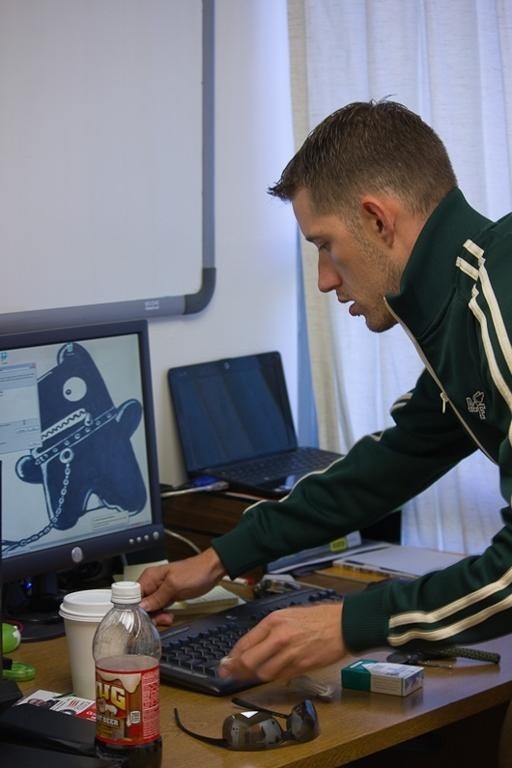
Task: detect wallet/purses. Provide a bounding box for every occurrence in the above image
[1,703,97,756]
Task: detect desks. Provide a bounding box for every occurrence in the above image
[0,540,512,767]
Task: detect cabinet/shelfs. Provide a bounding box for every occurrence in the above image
[162,480,402,561]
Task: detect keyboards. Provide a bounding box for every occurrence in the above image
[132,584,356,697]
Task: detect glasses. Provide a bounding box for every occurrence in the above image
[174,696,321,752]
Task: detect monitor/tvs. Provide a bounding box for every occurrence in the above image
[0,318,167,641]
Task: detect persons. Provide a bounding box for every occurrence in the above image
[28,696,60,709]
[137,101,511,682]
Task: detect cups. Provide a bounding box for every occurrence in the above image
[59,588,110,702]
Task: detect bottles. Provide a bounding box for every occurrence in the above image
[91,578,166,768]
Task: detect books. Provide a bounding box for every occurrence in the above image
[264,529,467,586]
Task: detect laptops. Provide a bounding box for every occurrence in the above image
[167,351,348,499]
[333,544,467,584]
